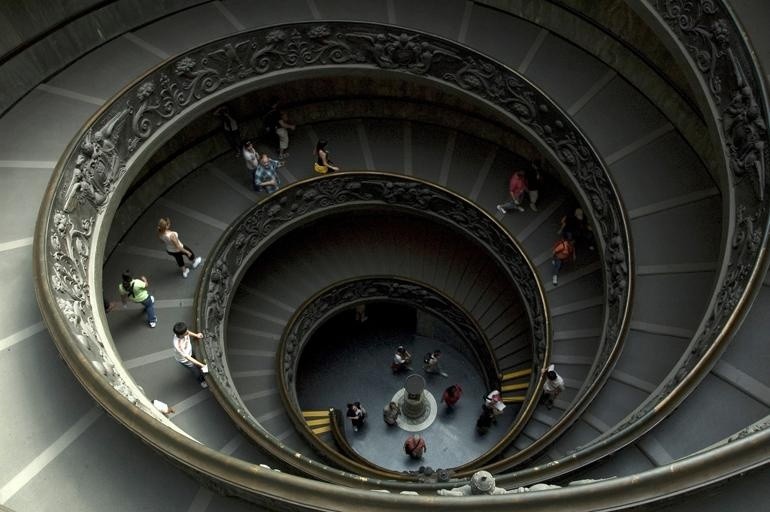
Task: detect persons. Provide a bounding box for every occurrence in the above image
[551,207,596,285]
[118,269,158,328]
[212,105,243,159]
[475,389,504,436]
[382,400,402,427]
[388,345,415,375]
[439,384,464,414]
[312,138,340,173]
[156,217,202,279]
[523,158,544,213]
[403,434,428,460]
[274,109,297,160]
[170,322,210,389]
[253,153,286,196]
[539,364,565,410]
[262,96,281,133]
[496,167,529,216]
[421,350,449,377]
[346,401,367,433]
[241,140,265,192]
[353,304,370,324]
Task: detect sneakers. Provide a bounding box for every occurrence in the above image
[552,274,558,285]
[496,204,506,214]
[517,205,525,212]
[149,316,157,328]
[150,295,155,303]
[192,257,201,268]
[182,268,190,278]
[200,380,208,389]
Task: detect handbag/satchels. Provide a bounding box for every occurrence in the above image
[314,163,329,174]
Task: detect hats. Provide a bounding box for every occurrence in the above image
[575,208,584,220]
